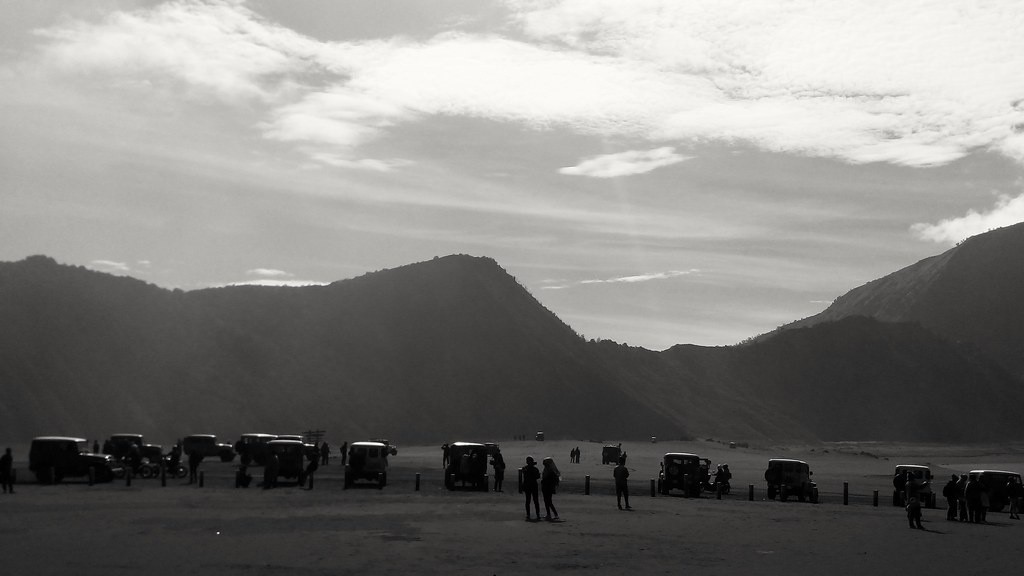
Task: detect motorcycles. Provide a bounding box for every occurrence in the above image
[152,456,188,478]
[114,456,153,480]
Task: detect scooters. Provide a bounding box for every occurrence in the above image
[700,470,731,495]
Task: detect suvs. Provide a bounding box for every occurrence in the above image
[444,441,488,491]
[969,469,1024,512]
[184,435,233,463]
[344,442,388,487]
[658,453,704,496]
[263,440,304,489]
[234,433,315,466]
[109,434,163,462]
[535,431,545,441]
[602,445,620,465]
[764,458,818,503]
[485,443,494,455]
[892,464,934,508]
[28,436,114,483]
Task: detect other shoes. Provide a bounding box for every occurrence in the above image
[544,515,552,520]
[1016,514,1020,520]
[917,525,925,530]
[910,525,916,529]
[625,505,632,508]
[553,517,559,520]
[1010,516,1016,519]
[618,506,622,510]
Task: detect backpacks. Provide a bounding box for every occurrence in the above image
[943,484,950,497]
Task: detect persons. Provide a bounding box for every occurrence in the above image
[0,447,16,494]
[1006,476,1020,520]
[320,442,330,465]
[93,439,109,453]
[522,456,541,519]
[167,439,182,477]
[614,457,632,510]
[541,457,561,519]
[946,472,989,524]
[131,443,140,477]
[188,447,199,485]
[303,442,320,483]
[489,449,506,492]
[441,443,449,469]
[340,442,347,465]
[904,471,926,530]
[621,452,627,461]
[716,463,729,482]
[570,447,580,463]
